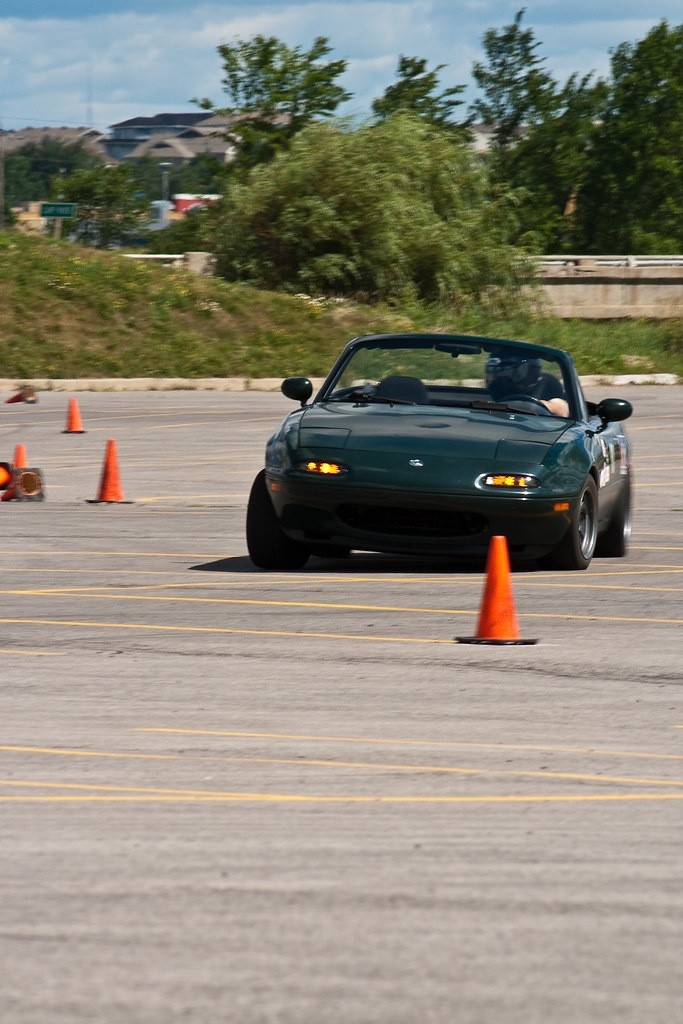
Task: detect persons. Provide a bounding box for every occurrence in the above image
[490,353,569,417]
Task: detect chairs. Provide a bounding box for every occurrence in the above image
[375,376,429,405]
[538,374,562,399]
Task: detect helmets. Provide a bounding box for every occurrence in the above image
[486,351,542,406]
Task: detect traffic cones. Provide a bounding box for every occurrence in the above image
[84,439,134,504]
[453,536,540,646]
[65,397,84,433]
[0,444,45,502]
[7,387,34,404]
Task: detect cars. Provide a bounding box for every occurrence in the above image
[246,333,632,570]
